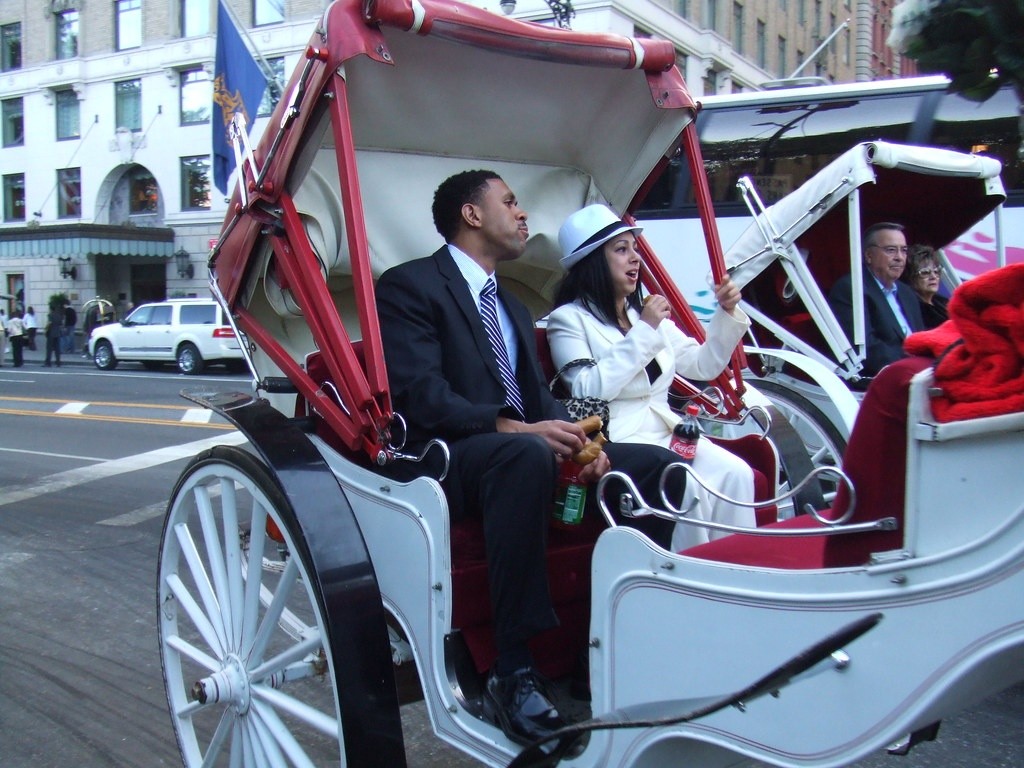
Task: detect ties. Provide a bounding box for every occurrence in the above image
[480,279,526,422]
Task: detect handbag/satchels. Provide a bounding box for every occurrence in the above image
[550,359,610,438]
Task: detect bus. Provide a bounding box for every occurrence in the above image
[535,68,1024,328]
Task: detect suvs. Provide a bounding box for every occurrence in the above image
[88,298,250,376]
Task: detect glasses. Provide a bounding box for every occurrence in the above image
[915,267,942,280]
[866,242,909,256]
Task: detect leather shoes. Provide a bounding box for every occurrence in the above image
[486,662,574,746]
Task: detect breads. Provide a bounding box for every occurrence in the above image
[572,415,604,464]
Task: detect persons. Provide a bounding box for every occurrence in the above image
[375,172,690,759]
[824,221,950,392]
[547,203,758,554]
[0,301,135,368]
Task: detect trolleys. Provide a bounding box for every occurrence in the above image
[80,295,117,329]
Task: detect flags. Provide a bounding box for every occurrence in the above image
[212,1,268,195]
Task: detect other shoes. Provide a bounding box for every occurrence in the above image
[40,364,50,368]
[55,364,60,368]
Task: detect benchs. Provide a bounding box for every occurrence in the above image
[246,271,781,674]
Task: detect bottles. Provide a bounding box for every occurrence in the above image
[668,405,700,466]
[551,453,588,532]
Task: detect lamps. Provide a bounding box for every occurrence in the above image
[56,255,79,280]
[170,246,194,281]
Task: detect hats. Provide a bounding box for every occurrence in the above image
[557,203,643,272]
